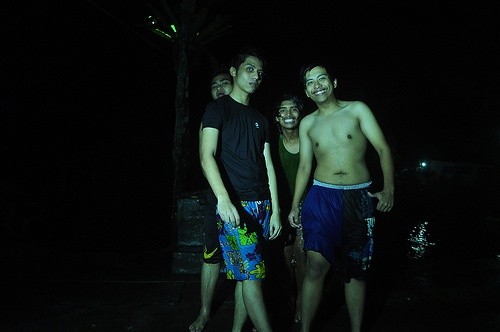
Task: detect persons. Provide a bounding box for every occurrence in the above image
[273,95,318,326]
[198,53,284,332]
[286,64,396,332]
[186,68,250,332]
